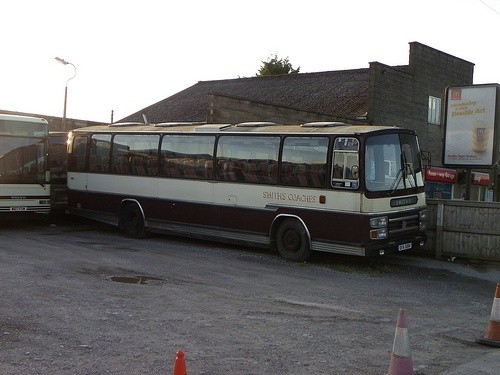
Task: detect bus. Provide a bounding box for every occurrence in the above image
[65,112,430,263]
[46,130,77,224]
[0,111,52,224]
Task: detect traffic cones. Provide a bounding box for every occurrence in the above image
[386,308,423,375]
[171,348,187,375]
[476,282,500,347]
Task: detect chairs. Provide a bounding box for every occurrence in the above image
[73,155,349,188]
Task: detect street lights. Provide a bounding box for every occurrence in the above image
[54,56,78,131]
